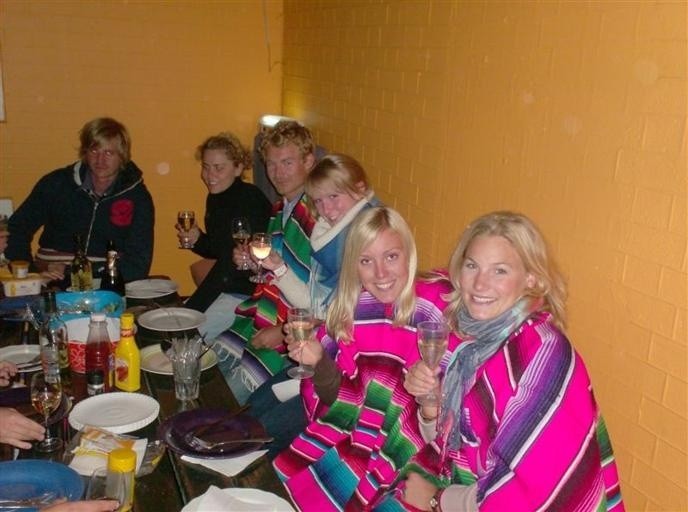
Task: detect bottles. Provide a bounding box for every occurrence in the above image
[70,235,125,296]
[11,261,30,278]
[39,291,141,394]
[109,448,136,512]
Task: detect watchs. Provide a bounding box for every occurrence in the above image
[430,487,444,511]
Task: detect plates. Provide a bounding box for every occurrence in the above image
[0,344,43,368]
[66,278,102,292]
[159,406,296,460]
[0,459,85,512]
[18,365,43,373]
[180,487,297,512]
[0,387,73,426]
[68,391,161,435]
[124,279,217,376]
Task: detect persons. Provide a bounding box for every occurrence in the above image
[1,225,9,255]
[2,458,120,511]
[175,133,273,295]
[240,152,390,459]
[0,361,46,450]
[376,211,626,512]
[197,118,333,402]
[270,206,455,512]
[7,118,155,291]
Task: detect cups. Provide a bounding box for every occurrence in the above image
[172,352,201,400]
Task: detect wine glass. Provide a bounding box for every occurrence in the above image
[232,218,271,284]
[178,211,194,249]
[85,468,125,512]
[0,219,10,266]
[30,371,63,452]
[286,308,315,380]
[416,321,448,407]
